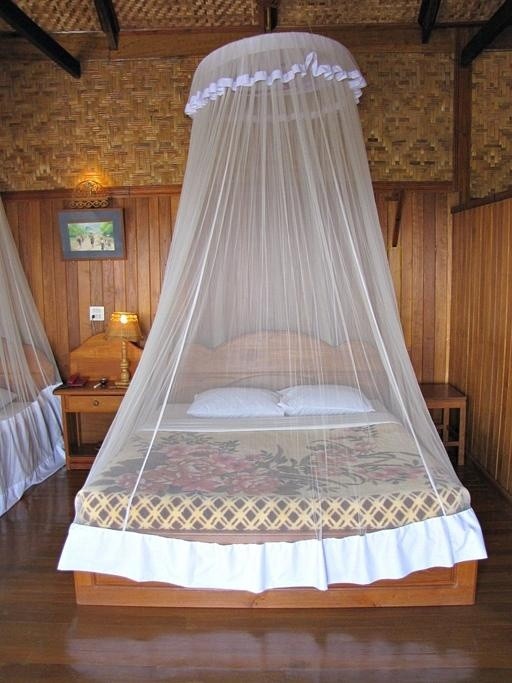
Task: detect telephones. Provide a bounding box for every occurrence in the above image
[66,373,90,387]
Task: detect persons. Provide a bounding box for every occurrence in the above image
[105,239,107,245]
[88,230,96,247]
[101,237,105,249]
[107,238,113,247]
[76,233,85,247]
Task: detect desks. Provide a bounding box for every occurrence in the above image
[414,379,469,465]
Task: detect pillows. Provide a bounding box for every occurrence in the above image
[187,387,285,420]
[1,386,15,407]
[277,384,377,417]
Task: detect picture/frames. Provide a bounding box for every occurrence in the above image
[58,207,128,262]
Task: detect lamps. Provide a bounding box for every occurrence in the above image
[107,310,142,388]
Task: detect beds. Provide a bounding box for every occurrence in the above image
[0,333,72,524]
[57,326,489,610]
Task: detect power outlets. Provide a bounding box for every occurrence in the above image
[89,305,105,320]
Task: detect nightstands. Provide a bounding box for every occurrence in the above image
[51,382,128,477]
